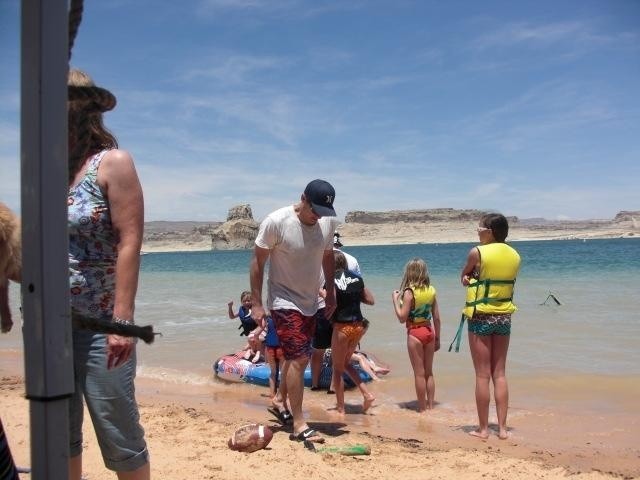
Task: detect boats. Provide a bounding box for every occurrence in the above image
[213,354,373,386]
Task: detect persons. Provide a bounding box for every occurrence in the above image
[67,73,165,480]
[463,213,521,437]
[392,259,443,414]
[250,179,338,440]
[228,232,389,412]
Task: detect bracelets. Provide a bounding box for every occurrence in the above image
[111,319,137,326]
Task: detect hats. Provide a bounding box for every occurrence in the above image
[304,179,337,216]
[69,68,117,112]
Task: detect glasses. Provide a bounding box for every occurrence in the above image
[310,202,318,215]
[477,225,492,234]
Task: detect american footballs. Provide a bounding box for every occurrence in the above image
[228,424,273,454]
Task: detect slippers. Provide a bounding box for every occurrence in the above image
[267,406,293,425]
[294,428,324,442]
